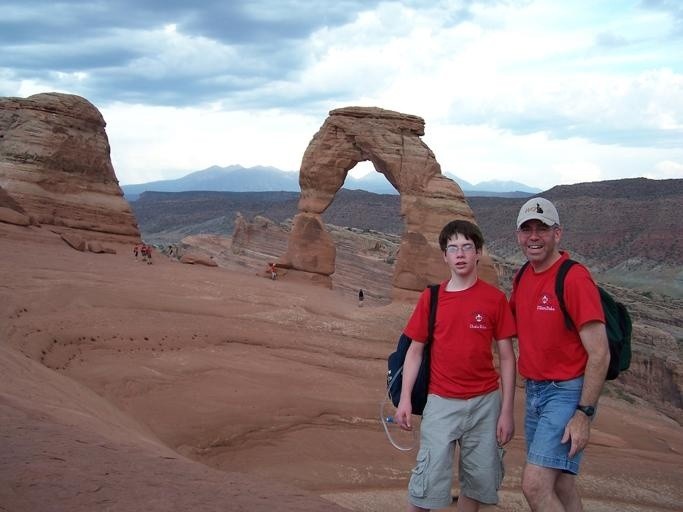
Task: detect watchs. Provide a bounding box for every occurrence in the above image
[577,404,598,420]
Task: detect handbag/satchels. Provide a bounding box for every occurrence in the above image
[386,282,441,415]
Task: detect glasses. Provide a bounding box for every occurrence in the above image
[444,244,478,254]
[518,227,561,234]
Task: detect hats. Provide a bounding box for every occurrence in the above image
[516,197,560,227]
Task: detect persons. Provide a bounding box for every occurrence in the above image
[133,241,153,264]
[358,288,364,300]
[168,244,174,261]
[394,221,517,511]
[270,262,276,280]
[505,196,612,511]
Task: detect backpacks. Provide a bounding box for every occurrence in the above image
[516,259,633,380]
